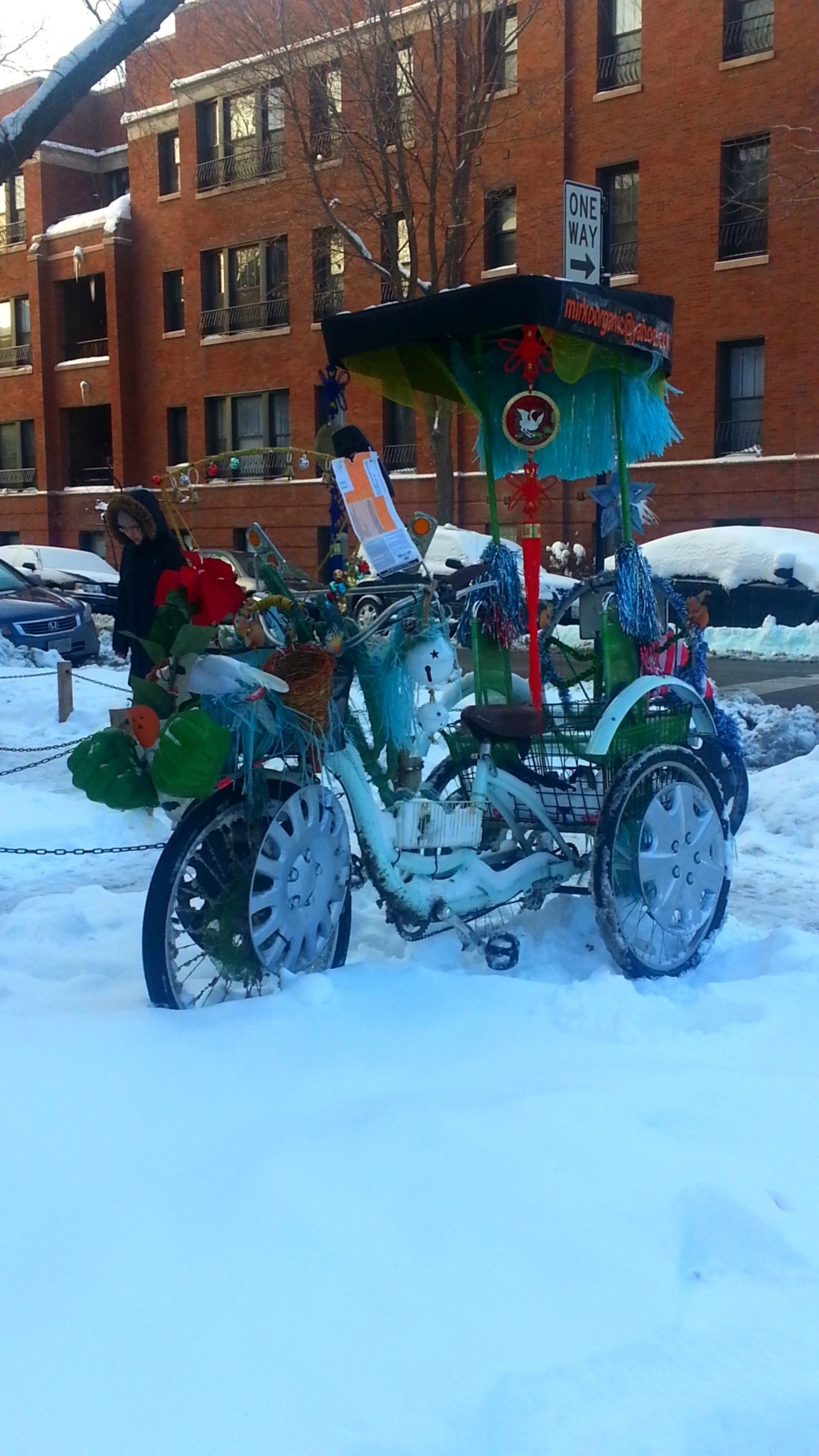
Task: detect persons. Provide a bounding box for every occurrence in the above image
[106,489,189,769]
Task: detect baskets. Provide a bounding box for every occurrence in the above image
[439,702,693,824]
[382,801,483,849]
[261,646,335,737]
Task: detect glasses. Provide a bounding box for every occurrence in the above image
[118,526,139,532]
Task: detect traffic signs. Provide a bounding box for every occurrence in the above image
[563,179,604,287]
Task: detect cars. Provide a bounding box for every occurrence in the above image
[0,558,101,669]
[605,523,819,630]
[0,543,122,617]
[192,545,337,623]
[342,521,584,643]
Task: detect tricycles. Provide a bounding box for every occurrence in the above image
[136,568,749,1018]
